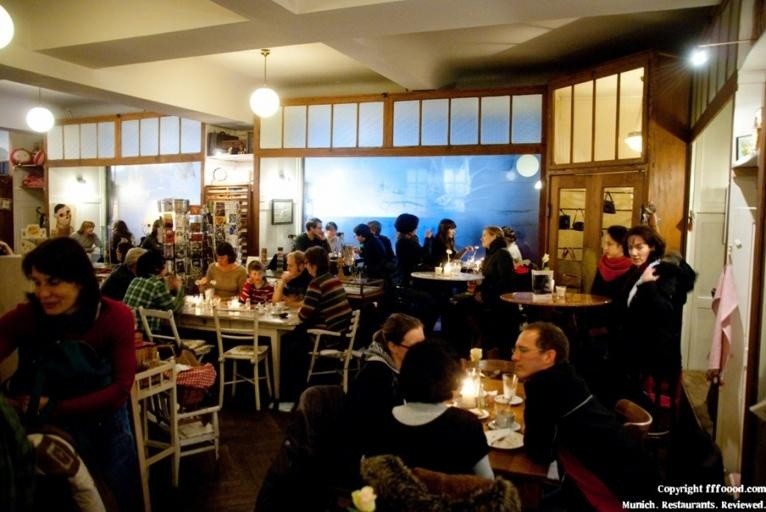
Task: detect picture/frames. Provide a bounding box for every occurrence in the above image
[271,198,293,224]
[736,134,755,160]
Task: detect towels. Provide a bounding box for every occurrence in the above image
[705,263,738,385]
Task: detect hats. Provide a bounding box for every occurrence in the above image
[395,214,418,234]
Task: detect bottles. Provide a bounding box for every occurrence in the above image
[245,298,250,310]
[261,247,267,262]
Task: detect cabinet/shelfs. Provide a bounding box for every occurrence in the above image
[203,185,252,275]
[13,164,45,189]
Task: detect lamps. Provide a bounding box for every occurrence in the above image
[626,76,642,152]
[249,48,280,118]
[27,87,54,133]
[692,39,751,66]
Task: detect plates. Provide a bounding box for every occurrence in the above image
[478,394,525,449]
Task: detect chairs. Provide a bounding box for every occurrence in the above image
[95,259,653,512]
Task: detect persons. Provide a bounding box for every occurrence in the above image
[1,213,698,512]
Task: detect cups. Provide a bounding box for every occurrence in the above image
[435,266,442,275]
[502,373,518,399]
[555,285,566,297]
